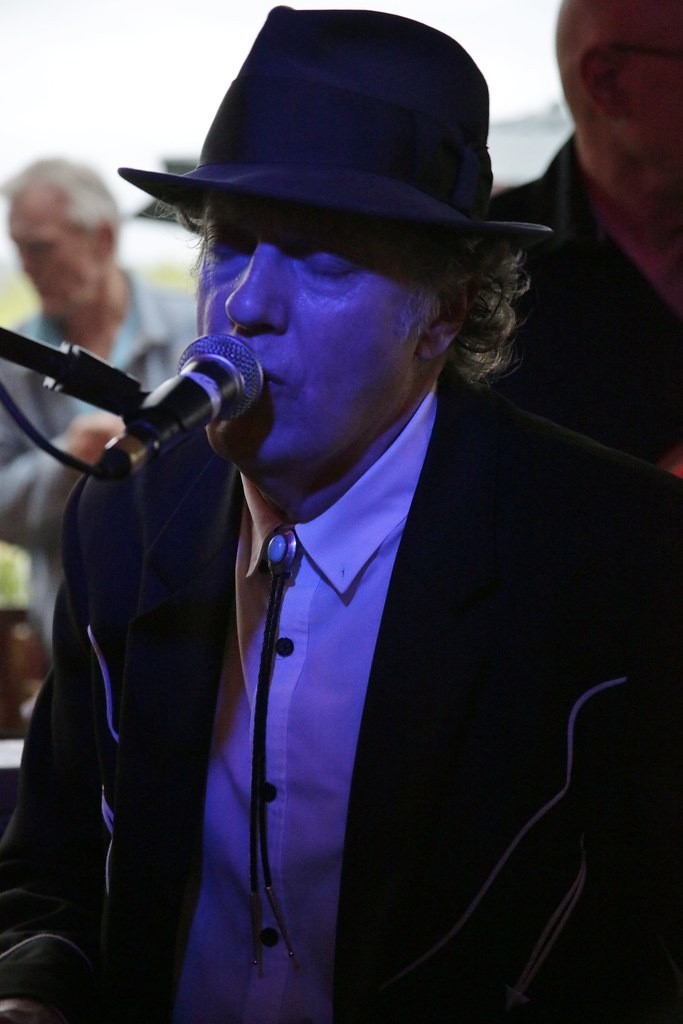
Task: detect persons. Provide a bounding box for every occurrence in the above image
[0,0,683,1024]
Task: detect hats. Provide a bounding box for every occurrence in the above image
[119,5,552,287]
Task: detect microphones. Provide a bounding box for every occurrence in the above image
[96,331,262,484]
